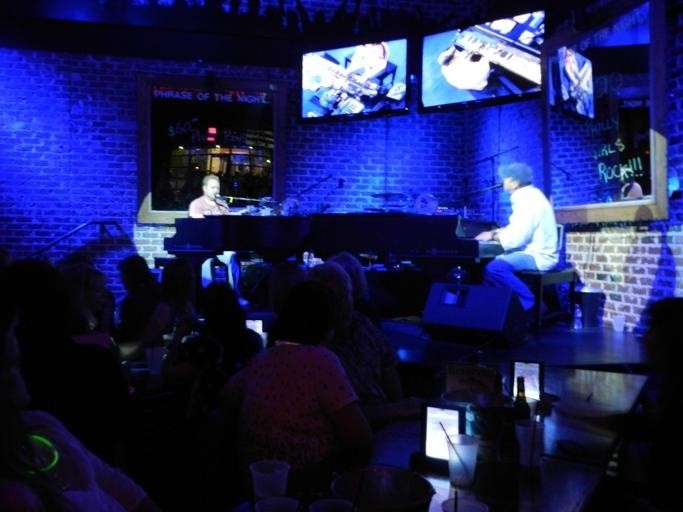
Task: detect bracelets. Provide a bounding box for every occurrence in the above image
[492,232,497,240]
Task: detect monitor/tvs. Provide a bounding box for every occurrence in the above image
[419,6,548,113]
[296,35,411,123]
[557,45,595,118]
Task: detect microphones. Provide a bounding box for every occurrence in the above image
[215,193,225,200]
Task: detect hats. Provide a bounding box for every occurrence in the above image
[497,163,535,184]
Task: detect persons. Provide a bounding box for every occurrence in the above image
[0,253,683,512]
[345,41,390,83]
[474,162,560,328]
[188,173,247,306]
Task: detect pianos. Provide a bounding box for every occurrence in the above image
[454,25,542,90]
[163,210,311,324]
[307,210,480,317]
[302,53,404,113]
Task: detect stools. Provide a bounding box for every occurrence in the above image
[522,267,575,334]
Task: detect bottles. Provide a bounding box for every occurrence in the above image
[512,376,531,425]
[573,304,583,330]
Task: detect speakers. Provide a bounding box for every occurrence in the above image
[420,282,517,347]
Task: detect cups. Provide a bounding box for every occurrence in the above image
[447,434,480,488]
[303,252,314,267]
